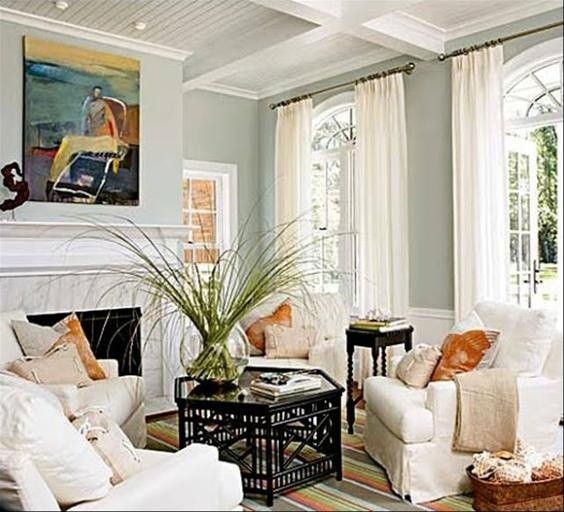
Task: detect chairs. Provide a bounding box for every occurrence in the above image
[0,370,244,511]
[230,292,349,411]
[362,303,563,506]
[0,308,147,447]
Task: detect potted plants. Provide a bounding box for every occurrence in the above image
[27,172,355,386]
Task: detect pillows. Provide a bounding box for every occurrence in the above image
[262,325,319,360]
[7,310,110,380]
[8,343,92,389]
[237,295,304,337]
[0,374,112,505]
[395,298,558,388]
[244,297,296,356]
[65,410,142,483]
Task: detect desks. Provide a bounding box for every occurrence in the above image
[342,325,413,434]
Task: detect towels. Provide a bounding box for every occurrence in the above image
[451,369,520,457]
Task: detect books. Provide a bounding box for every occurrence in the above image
[349,324,410,333]
[250,371,322,404]
[348,317,406,327]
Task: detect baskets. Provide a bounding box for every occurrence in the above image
[466,464,563,512]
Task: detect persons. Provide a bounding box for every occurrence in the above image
[80,86,118,139]
